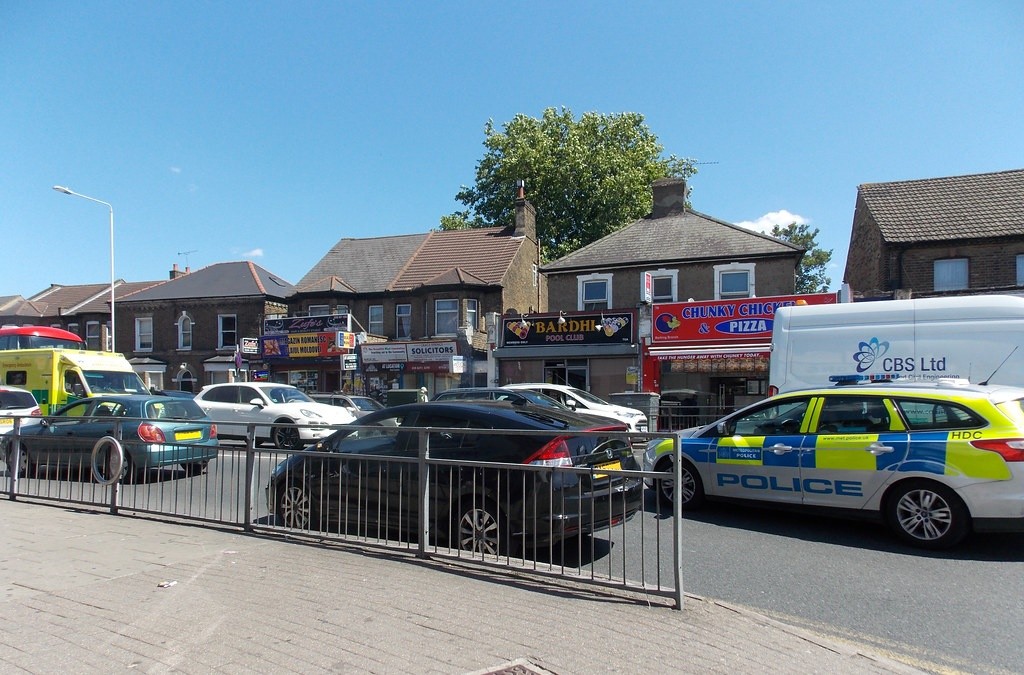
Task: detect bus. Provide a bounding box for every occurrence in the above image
[660,388,720,429]
[0,325,90,354]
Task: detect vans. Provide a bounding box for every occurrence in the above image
[767,293,1024,427]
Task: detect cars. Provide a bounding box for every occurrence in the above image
[153,389,198,400]
[495,381,649,445]
[307,392,399,440]
[0,393,220,485]
[635,371,1024,552]
[395,386,575,447]
[265,400,646,558]
[0,384,43,441]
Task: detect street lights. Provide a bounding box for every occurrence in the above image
[52,186,115,352]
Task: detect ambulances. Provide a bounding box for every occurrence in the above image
[0,347,164,425]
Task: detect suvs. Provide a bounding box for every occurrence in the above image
[187,381,359,452]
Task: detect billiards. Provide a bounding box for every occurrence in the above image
[507,322,531,339]
[601,317,629,336]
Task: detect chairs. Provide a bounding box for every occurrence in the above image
[91,405,112,422]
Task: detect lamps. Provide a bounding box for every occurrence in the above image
[594,313,606,332]
[520,313,528,327]
[558,309,566,323]
